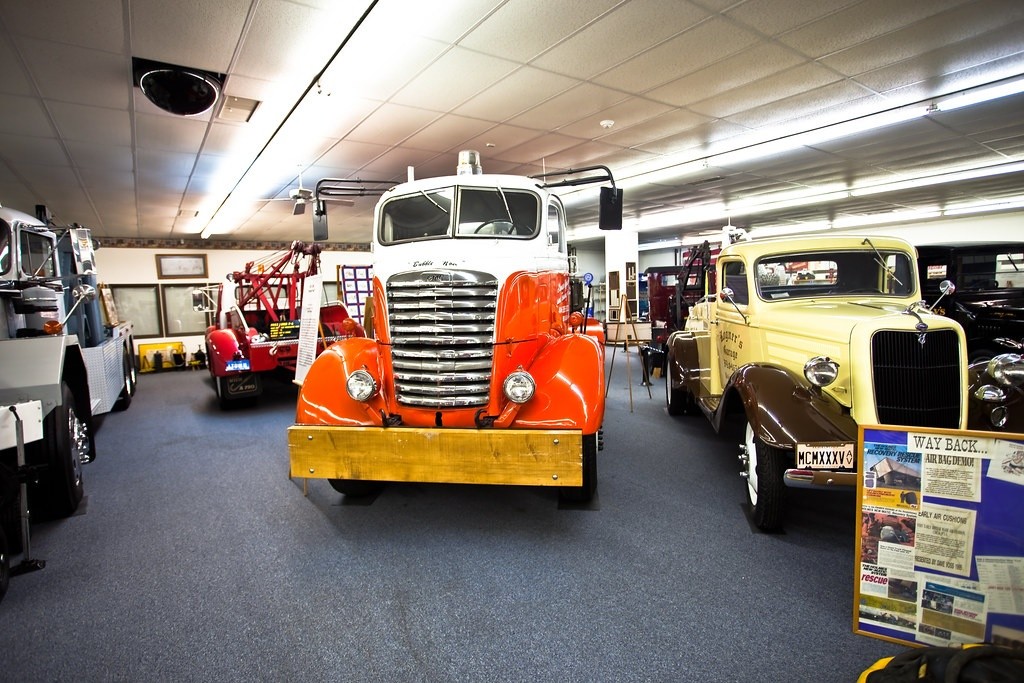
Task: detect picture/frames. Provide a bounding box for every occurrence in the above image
[155,254,208,279]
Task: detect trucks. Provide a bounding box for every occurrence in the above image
[284,148,624,503]
[193,242,366,412]
[647,240,717,377]
[0,206,136,509]
[894,242,1024,431]
[666,235,970,527]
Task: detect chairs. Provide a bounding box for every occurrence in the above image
[726,275,747,304]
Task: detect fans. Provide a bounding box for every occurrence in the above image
[259,163,354,215]
